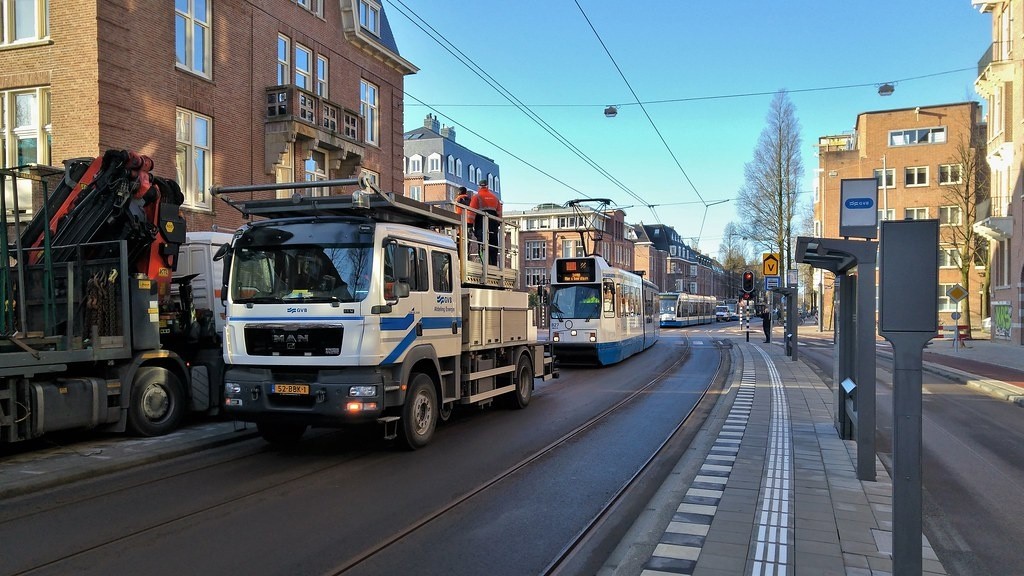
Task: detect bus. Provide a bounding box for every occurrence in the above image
[723,298,755,321]
[659,291,717,327]
[536,196,662,369]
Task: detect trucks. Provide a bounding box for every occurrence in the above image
[206,173,561,452]
[715,306,731,321]
[1,148,235,456]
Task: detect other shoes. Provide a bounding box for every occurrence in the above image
[763,341,769,343]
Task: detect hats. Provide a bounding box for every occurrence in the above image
[479,180,487,186]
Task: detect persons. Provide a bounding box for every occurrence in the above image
[758,308,771,344]
[579,291,600,304]
[777,308,782,320]
[358,250,395,301]
[455,187,473,260]
[466,179,502,264]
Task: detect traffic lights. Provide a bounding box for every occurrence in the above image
[741,270,755,293]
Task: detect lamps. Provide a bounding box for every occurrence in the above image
[604,105,617,117]
[877,83,894,95]
[829,170,838,177]
